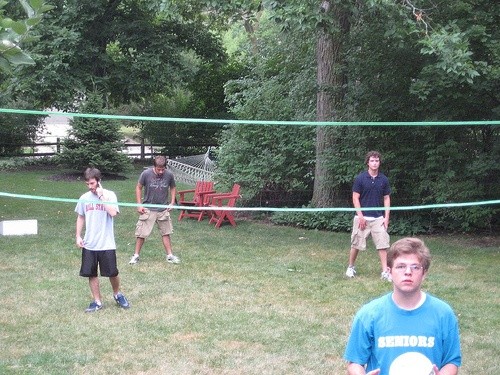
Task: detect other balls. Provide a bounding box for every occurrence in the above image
[388,351,434,375]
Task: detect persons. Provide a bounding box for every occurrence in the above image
[73,167,131,313]
[344,150,394,284]
[343,238,461,375]
[129,155,182,265]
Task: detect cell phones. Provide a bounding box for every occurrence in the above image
[97,180,99,187]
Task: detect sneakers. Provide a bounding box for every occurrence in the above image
[381,267,392,282]
[129,254,140,265]
[85,301,103,313]
[346,267,356,278]
[113,292,129,309]
[166,254,181,264]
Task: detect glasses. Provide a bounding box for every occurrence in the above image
[393,264,423,273]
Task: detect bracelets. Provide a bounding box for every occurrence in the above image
[97,194,102,199]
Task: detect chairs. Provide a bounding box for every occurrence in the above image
[178,181,241,228]
